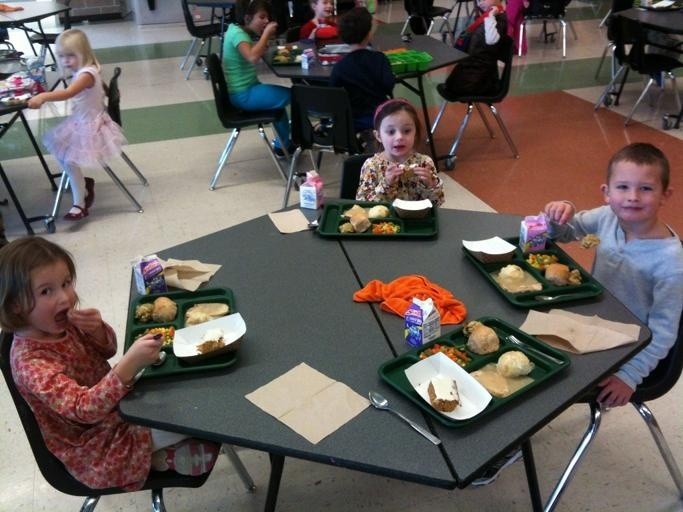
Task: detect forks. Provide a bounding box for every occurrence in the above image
[506,335,564,365]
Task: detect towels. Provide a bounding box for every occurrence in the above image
[243,361,370,444]
[268,208,312,234]
[164,258,222,293]
[351,274,466,325]
[519,308,641,355]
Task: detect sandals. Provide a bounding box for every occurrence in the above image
[84,177,94,208]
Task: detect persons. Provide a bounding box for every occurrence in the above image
[0,236,218,491]
[300,0,341,41]
[471,142,683,485]
[313,7,394,154]
[221,0,291,158]
[26,29,126,222]
[354,98,446,208]
[445,1,510,95]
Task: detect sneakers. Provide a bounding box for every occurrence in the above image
[275,139,300,159]
[62,205,88,220]
[471,442,525,487]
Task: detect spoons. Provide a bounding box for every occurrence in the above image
[308,205,324,228]
[368,391,442,445]
[153,332,162,340]
[536,292,594,302]
[125,351,166,388]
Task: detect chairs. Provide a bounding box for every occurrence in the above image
[205,53,293,192]
[594,18,681,127]
[30,0,72,61]
[343,159,372,198]
[519,0,577,58]
[425,36,519,169]
[44,66,148,233]
[284,84,371,212]
[178,1,228,80]
[539,240,683,511]
[401,0,452,40]
[2,325,256,511]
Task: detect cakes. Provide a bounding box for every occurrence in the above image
[196,321,228,353]
[397,162,419,179]
[428,379,461,413]
